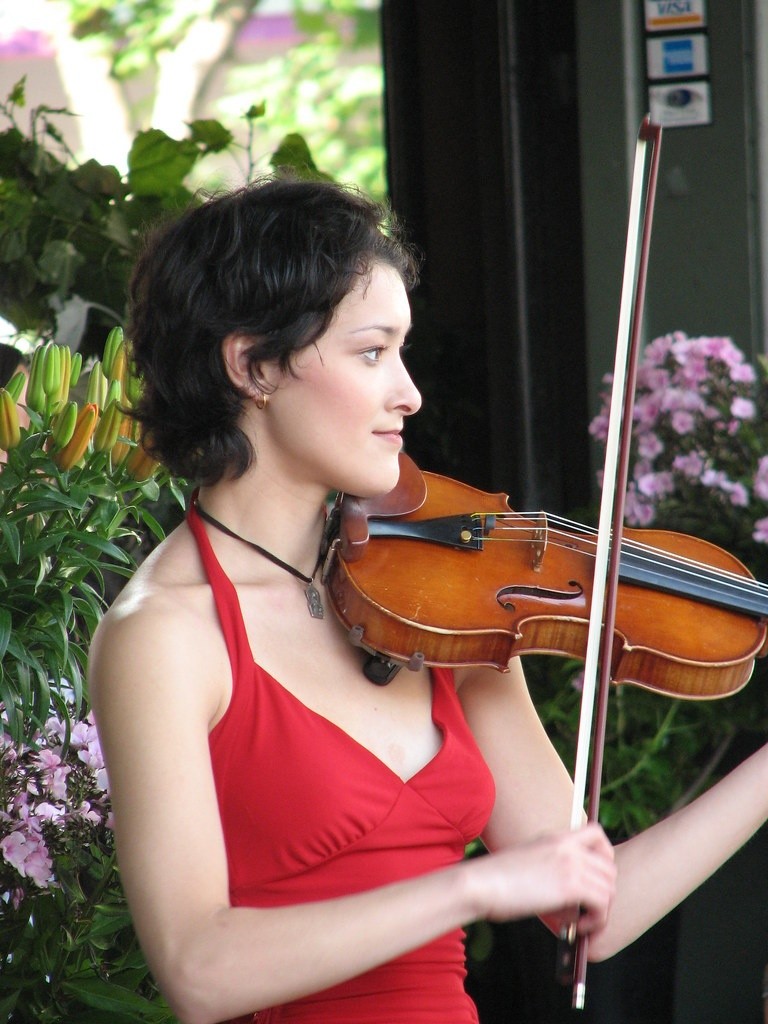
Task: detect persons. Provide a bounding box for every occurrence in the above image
[88,173,767,1024]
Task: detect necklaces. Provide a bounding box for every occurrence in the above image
[187,480,329,622]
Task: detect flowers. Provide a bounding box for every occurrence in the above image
[569,330,768,698]
[0,319,200,977]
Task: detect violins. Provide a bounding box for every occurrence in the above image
[332,465,768,703]
